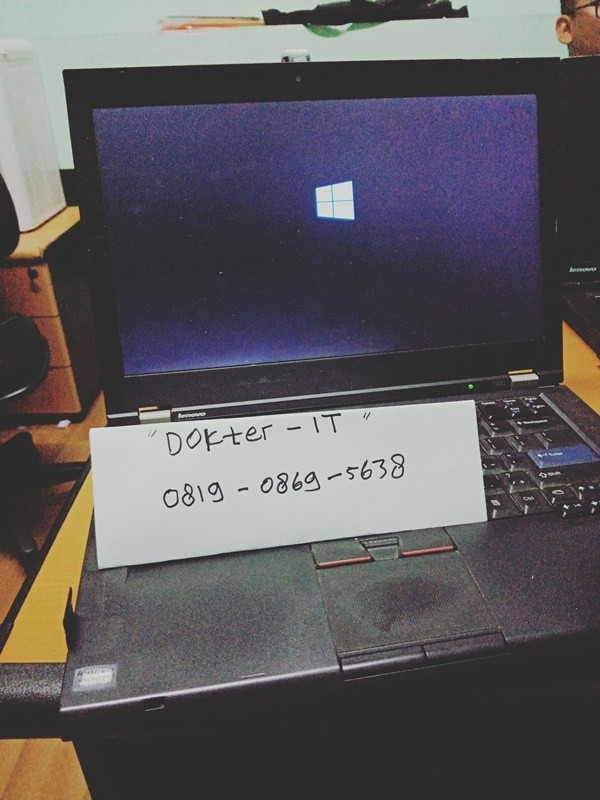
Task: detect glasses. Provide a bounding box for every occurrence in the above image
[575,0,600,18]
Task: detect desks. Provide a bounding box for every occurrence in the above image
[0,315,600,739]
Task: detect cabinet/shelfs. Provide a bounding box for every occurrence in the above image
[0,201,103,430]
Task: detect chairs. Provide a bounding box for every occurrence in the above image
[0,171,92,582]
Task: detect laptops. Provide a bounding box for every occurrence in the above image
[59,57,599,743]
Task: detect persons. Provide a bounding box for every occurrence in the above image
[555,0,600,59]
